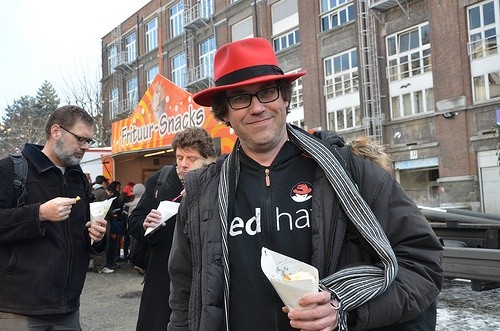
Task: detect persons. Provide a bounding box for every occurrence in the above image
[167,37,445,331]
[88,176,146,276]
[0,105,107,331]
[129,127,218,331]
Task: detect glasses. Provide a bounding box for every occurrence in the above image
[59,125,95,147]
[224,84,281,111]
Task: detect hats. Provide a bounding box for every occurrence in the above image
[191,37,308,106]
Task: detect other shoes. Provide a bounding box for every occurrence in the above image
[99,267,115,273]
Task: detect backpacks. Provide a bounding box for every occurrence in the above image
[126,166,173,268]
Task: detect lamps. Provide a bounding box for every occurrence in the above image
[442,112,455,118]
